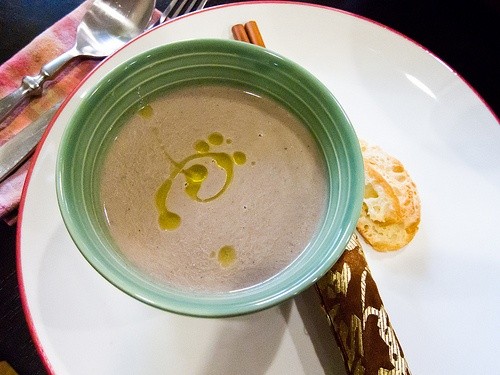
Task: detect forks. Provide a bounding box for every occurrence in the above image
[1,1,210,192]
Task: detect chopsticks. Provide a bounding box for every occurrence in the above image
[230,20,264,48]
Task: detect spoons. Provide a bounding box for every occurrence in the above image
[0,1,159,129]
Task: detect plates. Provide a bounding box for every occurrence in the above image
[17,0,499,373]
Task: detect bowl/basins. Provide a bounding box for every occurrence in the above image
[55,39,366,320]
